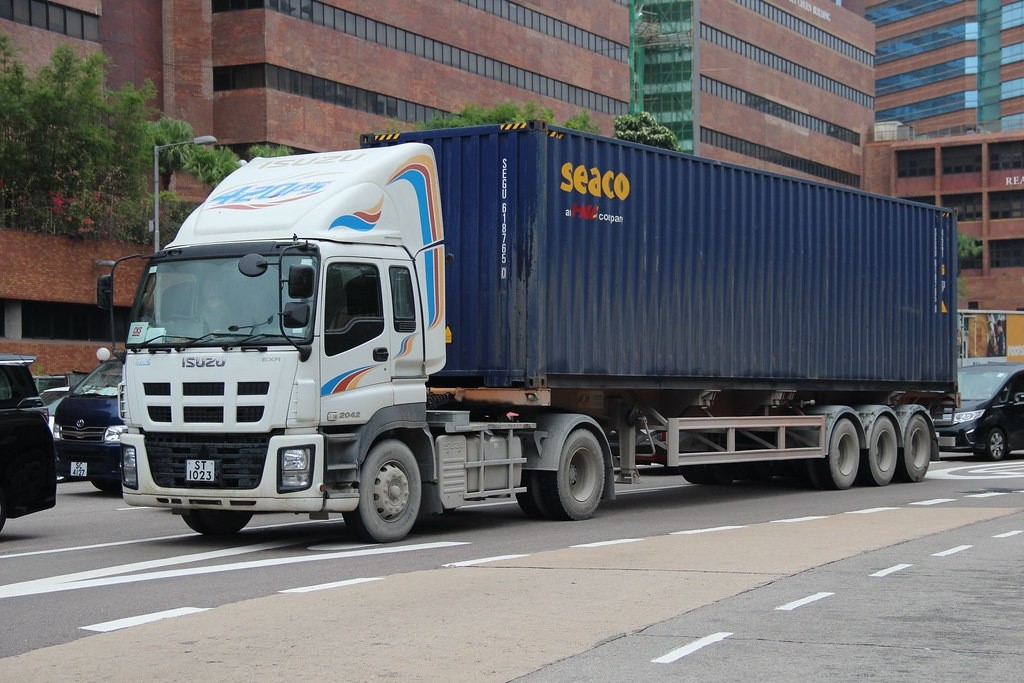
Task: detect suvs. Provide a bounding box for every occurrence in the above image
[0,350,59,536]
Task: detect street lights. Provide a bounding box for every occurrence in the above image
[149,135,219,254]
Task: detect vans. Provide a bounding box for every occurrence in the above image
[54,353,121,496]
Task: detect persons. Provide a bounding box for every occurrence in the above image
[200,279,228,331]
[996,320,1005,356]
[987,320,997,357]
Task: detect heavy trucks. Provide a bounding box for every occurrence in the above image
[119,120,957,540]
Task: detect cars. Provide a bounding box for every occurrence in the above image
[933,363,1024,459]
